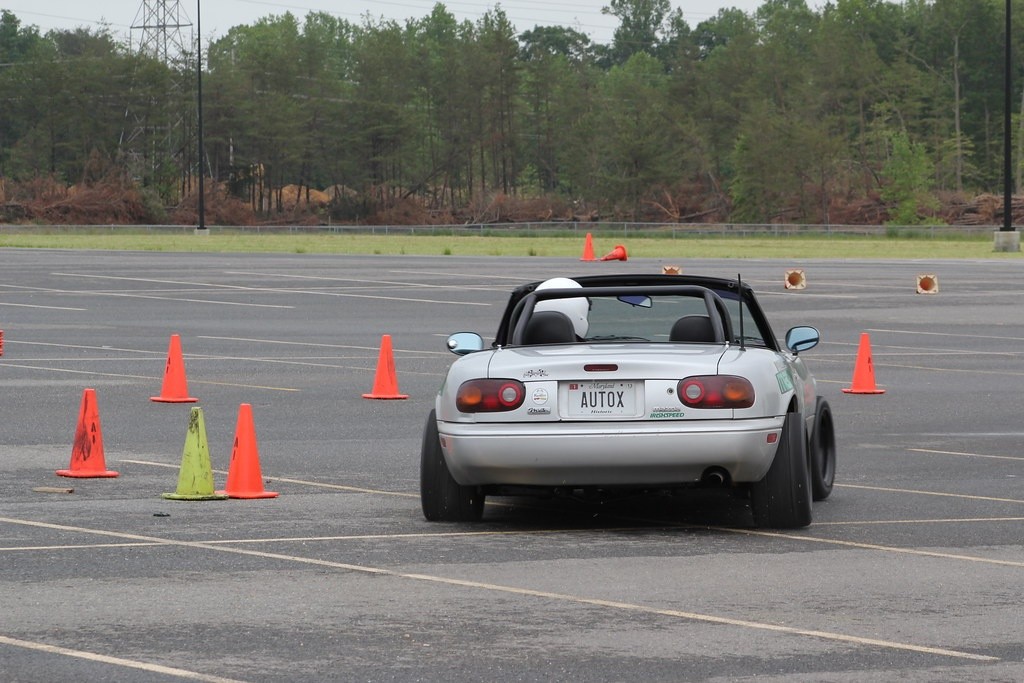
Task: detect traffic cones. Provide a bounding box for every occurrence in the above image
[55,390,119,478]
[600,245,629,262]
[841,333,886,394]
[163,406,232,500]
[150,334,199,402]
[784,269,807,290]
[661,265,682,276]
[212,402,281,499]
[361,335,411,400]
[579,233,597,262]
[916,274,939,295]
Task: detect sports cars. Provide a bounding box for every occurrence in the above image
[420,274,836,532]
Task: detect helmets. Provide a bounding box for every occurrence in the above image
[531,278,590,339]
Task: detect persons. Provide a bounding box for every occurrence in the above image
[530,277,591,343]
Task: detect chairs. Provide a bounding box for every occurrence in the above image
[668,314,719,343]
[518,311,577,344]
